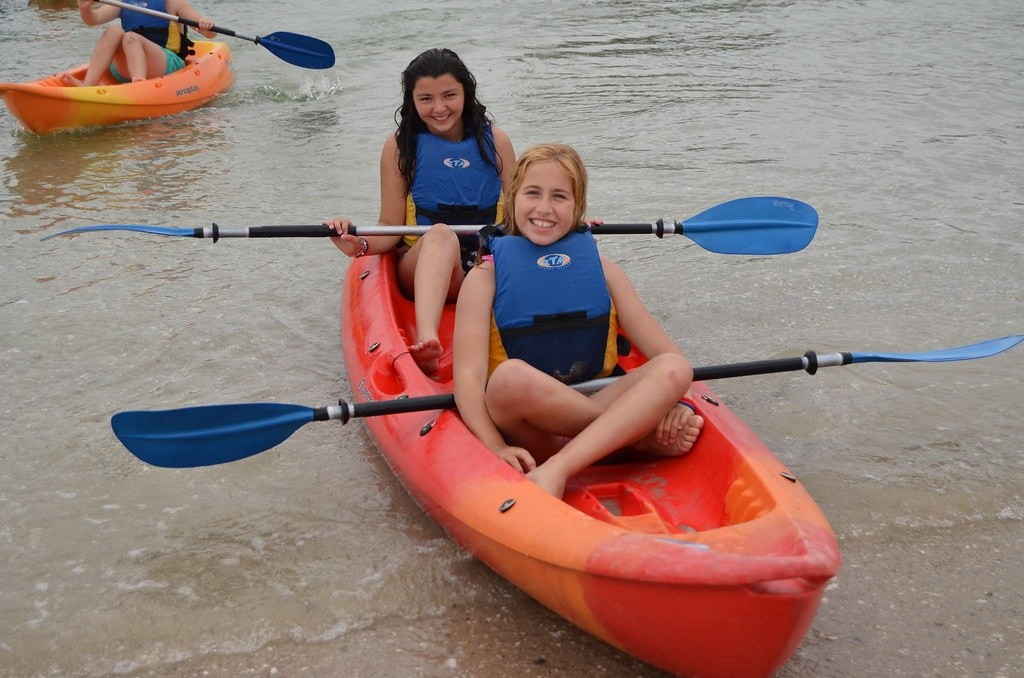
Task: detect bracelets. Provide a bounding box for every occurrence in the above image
[676,395,699,416]
[354,234,370,259]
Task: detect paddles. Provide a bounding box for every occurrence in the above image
[40,197,819,255]
[94,0,334,70]
[109,334,1023,468]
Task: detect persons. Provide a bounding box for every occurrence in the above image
[58,0,216,91]
[321,45,605,376]
[453,141,705,505]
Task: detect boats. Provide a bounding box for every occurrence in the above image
[340,241,843,678]
[0,38,236,136]
